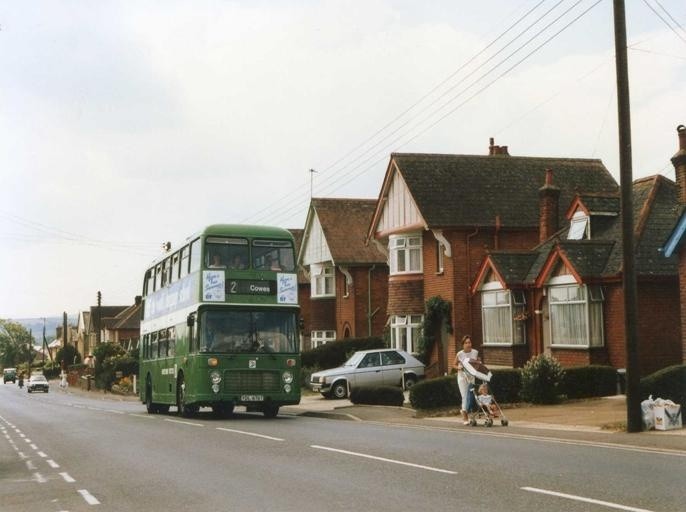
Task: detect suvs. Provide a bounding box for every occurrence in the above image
[3,368,16,384]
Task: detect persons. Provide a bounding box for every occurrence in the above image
[229,255,245,268]
[257,254,281,273]
[210,253,227,269]
[478,384,498,415]
[452,335,482,424]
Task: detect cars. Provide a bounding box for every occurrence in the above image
[27,375,50,393]
[310,348,426,399]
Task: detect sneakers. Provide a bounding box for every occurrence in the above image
[462,419,470,425]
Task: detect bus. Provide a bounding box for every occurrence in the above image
[136,223,301,417]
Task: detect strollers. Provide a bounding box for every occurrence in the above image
[461,366,508,427]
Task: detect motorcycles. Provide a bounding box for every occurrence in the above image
[18,376,24,389]
